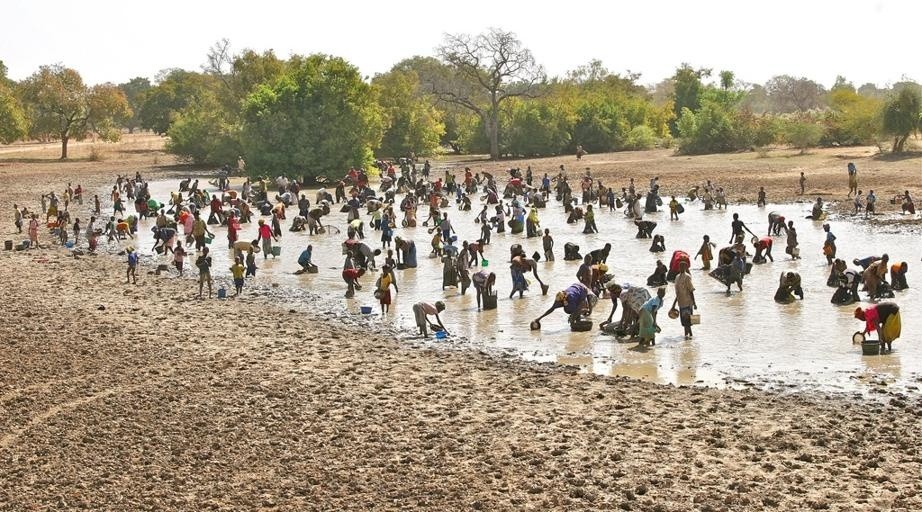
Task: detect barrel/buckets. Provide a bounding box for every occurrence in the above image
[4,177,902,360]
